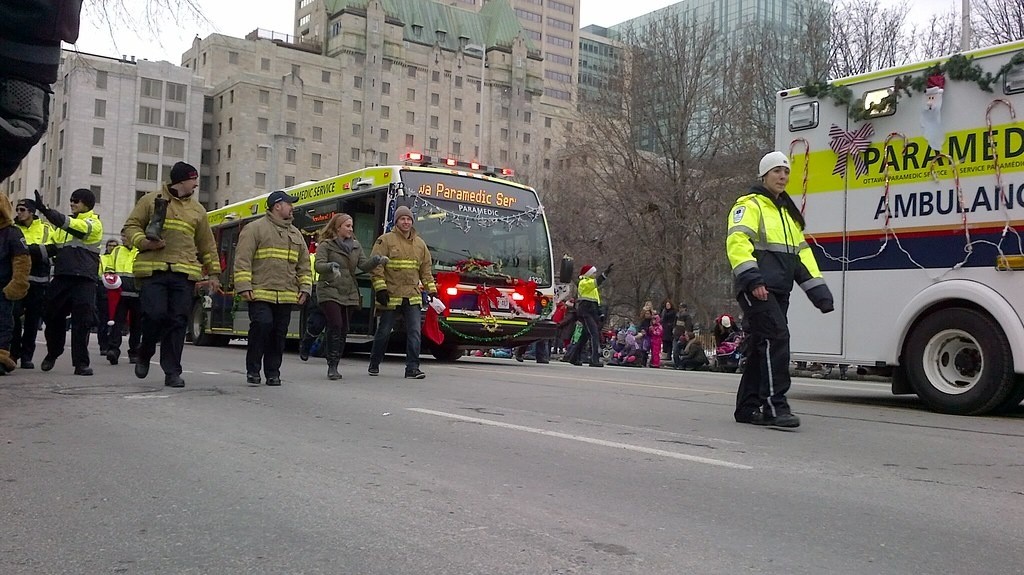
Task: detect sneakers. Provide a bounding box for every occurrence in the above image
[764,412,800,426]
[736,408,765,424]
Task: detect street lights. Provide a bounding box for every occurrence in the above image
[464,43,485,163]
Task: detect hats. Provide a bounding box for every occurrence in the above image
[578,265,597,278]
[758,150,792,178]
[678,302,687,307]
[106,237,118,247]
[171,161,198,183]
[267,191,300,207]
[628,326,636,332]
[722,316,731,328]
[394,206,412,217]
[16,198,36,213]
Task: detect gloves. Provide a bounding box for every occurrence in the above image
[34,189,49,215]
[429,292,439,303]
[376,289,390,307]
[602,263,614,278]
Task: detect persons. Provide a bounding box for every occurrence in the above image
[124,161,221,386]
[301,214,381,379]
[466,298,588,363]
[0,0,82,182]
[609,310,664,367]
[12,189,103,376]
[570,263,614,367]
[368,205,438,379]
[662,301,707,370]
[97,229,141,365]
[234,191,312,386]
[795,361,848,379]
[727,151,834,426]
[714,314,750,366]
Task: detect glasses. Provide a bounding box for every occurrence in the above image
[15,208,30,212]
[70,198,84,203]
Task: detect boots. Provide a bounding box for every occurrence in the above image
[146,199,169,240]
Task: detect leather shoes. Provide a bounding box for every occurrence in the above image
[10,349,185,387]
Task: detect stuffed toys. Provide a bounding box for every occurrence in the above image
[0,192,31,376]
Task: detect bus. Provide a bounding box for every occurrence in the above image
[185,152,573,360]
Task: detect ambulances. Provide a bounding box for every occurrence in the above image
[774,39,1024,416]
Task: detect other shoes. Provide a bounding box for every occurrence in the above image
[247,329,425,385]
[515,350,603,369]
